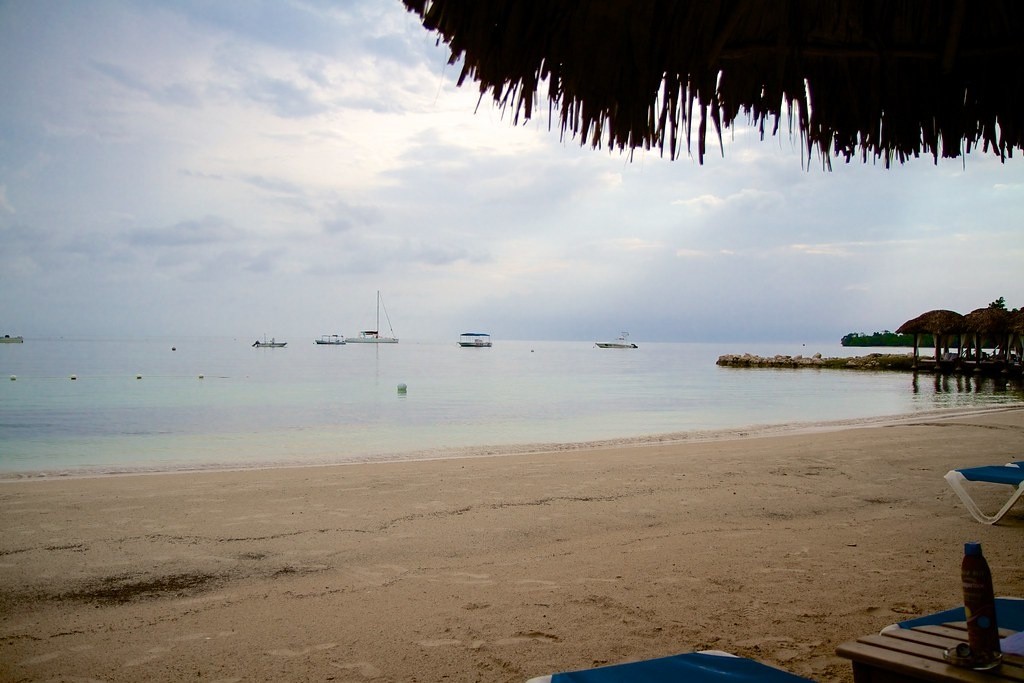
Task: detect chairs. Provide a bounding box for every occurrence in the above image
[523,651,815,683]
[943,462,1024,525]
[879,595,1024,633]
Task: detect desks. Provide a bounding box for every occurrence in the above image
[834,618,1024,683]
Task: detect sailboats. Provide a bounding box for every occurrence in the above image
[344,289,400,343]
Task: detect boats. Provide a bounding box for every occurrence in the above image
[0,335,24,343]
[252,331,288,347]
[314,334,346,344]
[457,332,492,347]
[595,342,638,348]
